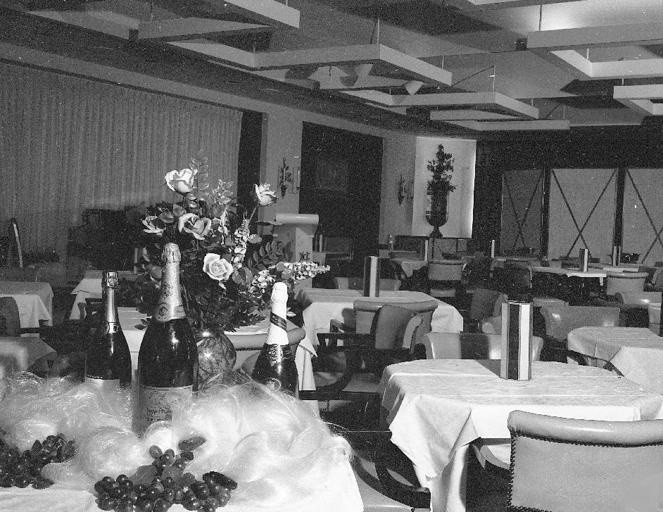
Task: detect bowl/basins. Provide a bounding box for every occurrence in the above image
[505,247,534,257]
[608,253,640,263]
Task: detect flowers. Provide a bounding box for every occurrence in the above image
[139,148,332,332]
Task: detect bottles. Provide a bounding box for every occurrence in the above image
[132,245,200,439]
[549,258,561,269]
[6,218,22,268]
[247,280,299,398]
[387,233,394,251]
[85,271,133,392]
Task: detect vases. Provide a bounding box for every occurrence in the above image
[187,326,238,397]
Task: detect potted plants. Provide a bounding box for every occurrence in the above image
[426,144,456,237]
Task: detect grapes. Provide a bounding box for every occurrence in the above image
[0,433,77,488]
[93,437,237,512]
